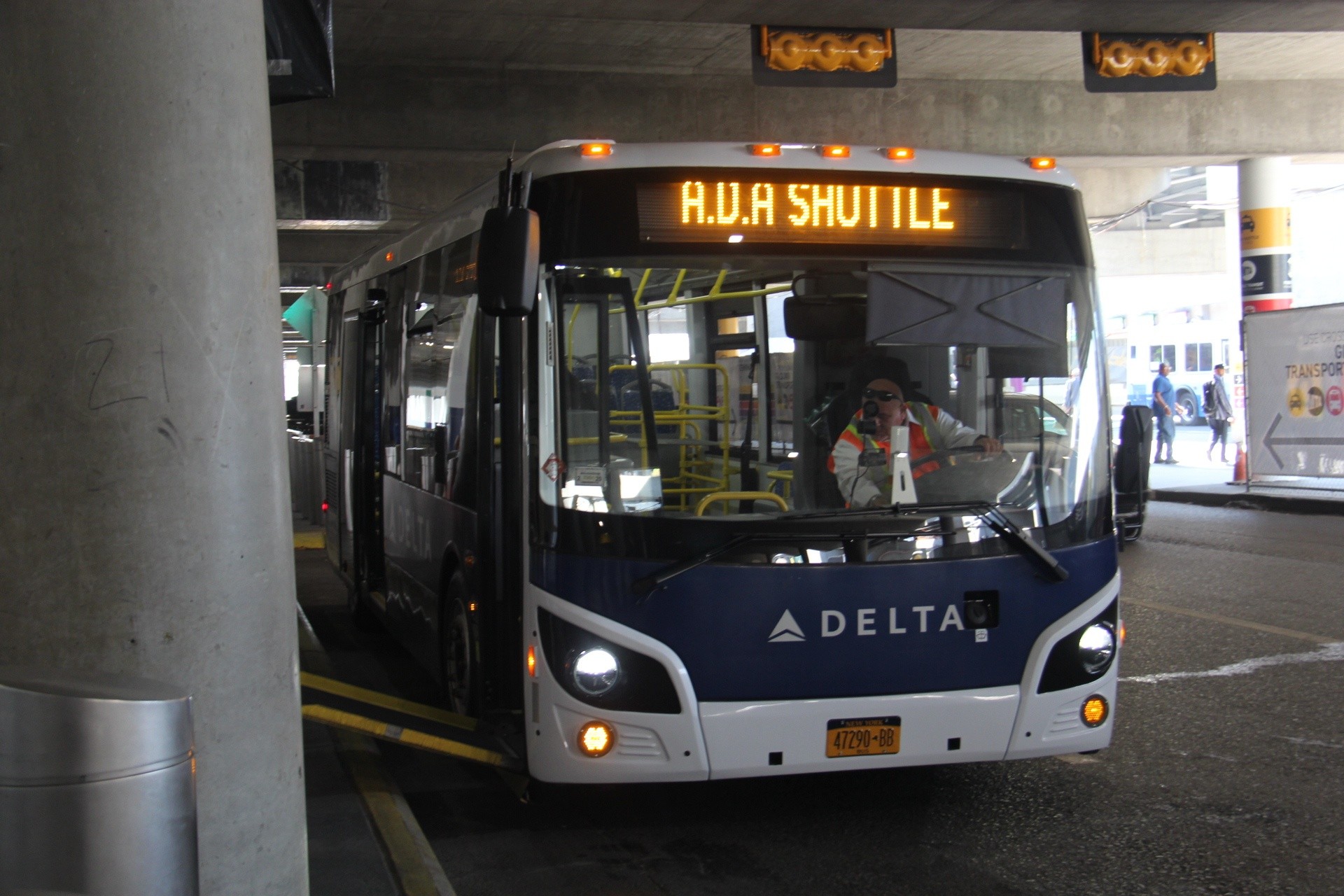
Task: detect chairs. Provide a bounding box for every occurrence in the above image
[810,353,937,508]
[562,351,678,435]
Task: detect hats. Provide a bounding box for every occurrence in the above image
[1215,363,1229,370]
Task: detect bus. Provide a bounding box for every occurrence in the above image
[317,131,1154,787]
[1124,318,1232,425]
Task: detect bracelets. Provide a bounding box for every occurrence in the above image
[1164,405,1168,409]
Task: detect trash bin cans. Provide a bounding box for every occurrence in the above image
[1,680,202,896]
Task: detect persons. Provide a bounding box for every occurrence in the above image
[833,380,1005,509]
[1205,364,1234,464]
[1152,363,1185,464]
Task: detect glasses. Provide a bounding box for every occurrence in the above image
[863,385,902,405]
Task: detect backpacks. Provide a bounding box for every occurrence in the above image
[1202,381,1217,416]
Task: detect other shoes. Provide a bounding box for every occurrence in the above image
[1153,458,1164,463]
[1166,459,1178,464]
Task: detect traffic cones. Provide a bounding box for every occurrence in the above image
[1226,442,1247,486]
